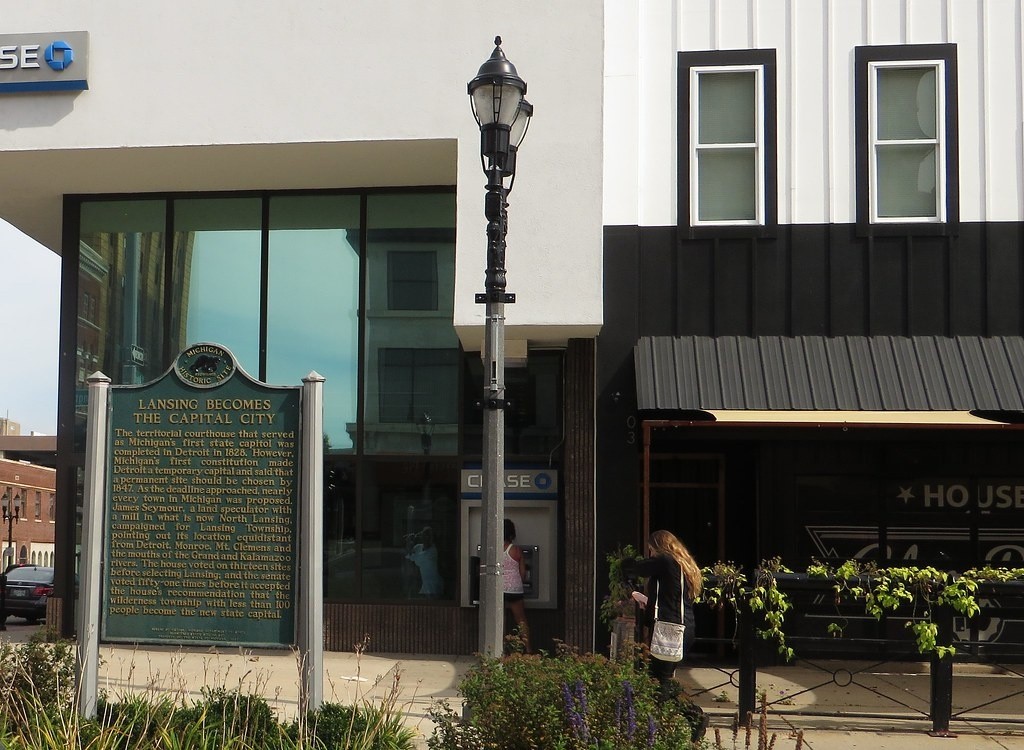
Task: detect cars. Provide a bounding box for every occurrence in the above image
[5,566,79,625]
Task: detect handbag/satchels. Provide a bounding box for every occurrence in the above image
[649,621,686,663]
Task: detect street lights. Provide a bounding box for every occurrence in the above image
[465,37,534,683]
[0,490,21,568]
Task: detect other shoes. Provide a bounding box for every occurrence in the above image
[691,714,709,743]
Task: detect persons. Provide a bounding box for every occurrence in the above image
[503,518,532,654]
[626,532,708,740]
[404,528,445,598]
